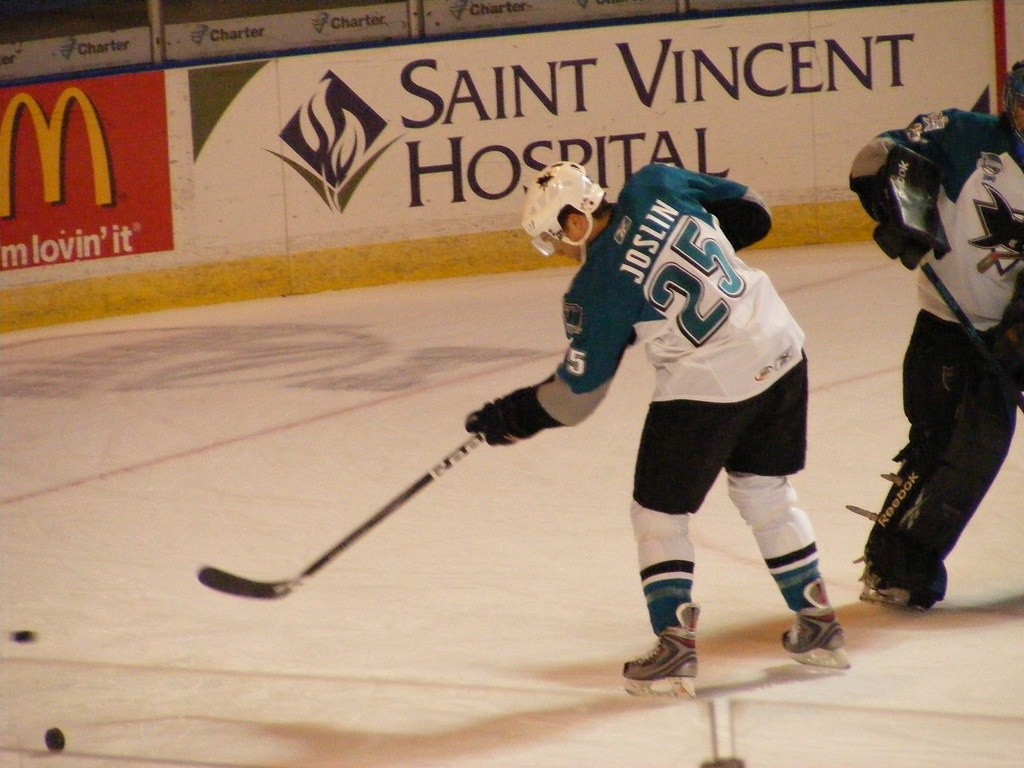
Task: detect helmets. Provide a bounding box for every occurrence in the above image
[1005,59,1024,110]
[522,161,606,245]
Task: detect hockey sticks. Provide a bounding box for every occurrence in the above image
[195,425,486,604]
[921,258,1024,418]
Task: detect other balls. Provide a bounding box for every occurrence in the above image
[44,726,66,753]
[13,630,35,643]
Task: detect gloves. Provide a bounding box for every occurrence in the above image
[464,386,566,447]
[900,226,936,271]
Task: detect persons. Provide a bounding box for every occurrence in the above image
[846,58,1024,610]
[460,156,851,700]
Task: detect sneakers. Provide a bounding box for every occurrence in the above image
[621,603,700,699]
[782,579,854,671]
